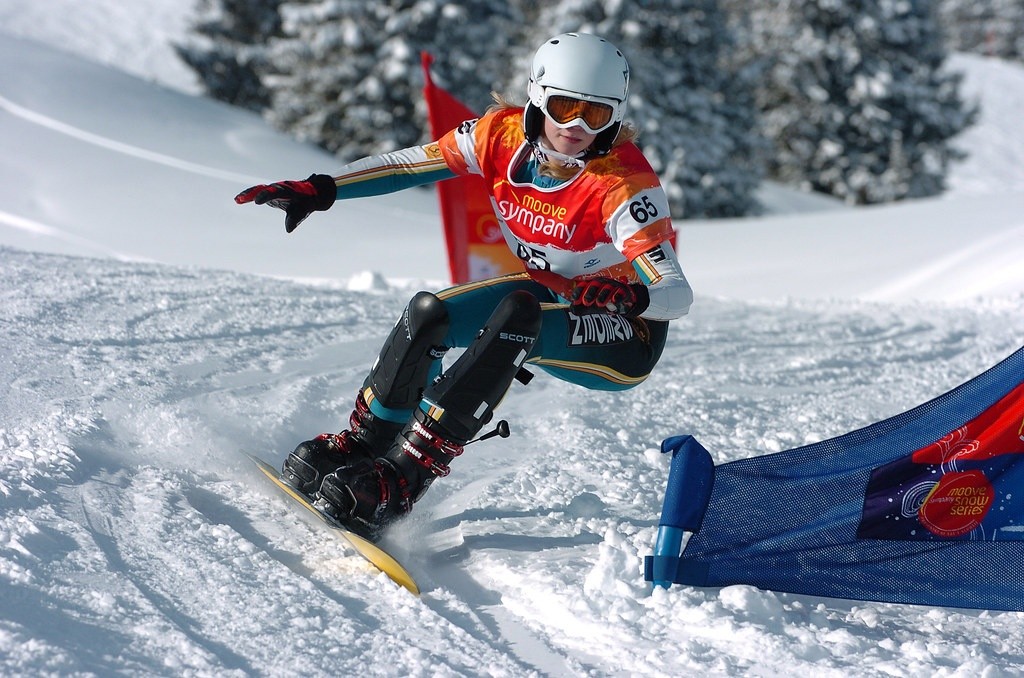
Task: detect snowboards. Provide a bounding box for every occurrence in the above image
[246,453,422,594]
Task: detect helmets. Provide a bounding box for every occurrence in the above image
[523,32,630,156]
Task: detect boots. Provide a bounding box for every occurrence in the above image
[314,407,472,543]
[281,388,409,495]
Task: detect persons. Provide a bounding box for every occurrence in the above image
[235,30,694,543]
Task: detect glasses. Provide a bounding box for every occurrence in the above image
[526,81,627,134]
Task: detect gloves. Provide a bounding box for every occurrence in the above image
[234,174,337,234]
[569,276,651,320]
